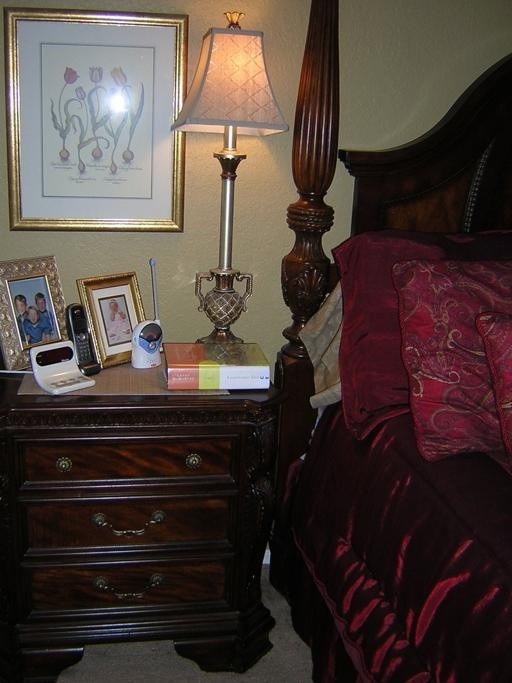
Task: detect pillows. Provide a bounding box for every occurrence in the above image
[392,257,509,463]
[330,227,510,464]
[474,310,511,463]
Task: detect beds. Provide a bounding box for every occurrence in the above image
[268,53,512,682]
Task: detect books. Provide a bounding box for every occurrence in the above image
[162,342,271,390]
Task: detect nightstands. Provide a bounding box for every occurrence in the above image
[0,353,289,683]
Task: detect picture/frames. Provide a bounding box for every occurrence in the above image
[4,7,191,233]
[1,255,147,371]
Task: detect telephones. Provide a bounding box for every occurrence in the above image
[65,303,101,375]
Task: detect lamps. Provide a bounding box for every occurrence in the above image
[170,10,291,343]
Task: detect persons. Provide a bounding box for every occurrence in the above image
[106,300,131,339]
[13,292,56,345]
